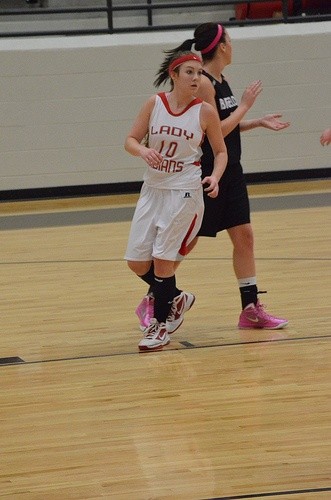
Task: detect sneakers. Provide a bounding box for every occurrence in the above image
[136,295,156,330]
[136,318,170,351]
[166,290,196,334]
[238,298,290,330]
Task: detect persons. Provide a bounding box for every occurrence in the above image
[135,22,290,331]
[123,51,229,350]
[319,127,331,146]
